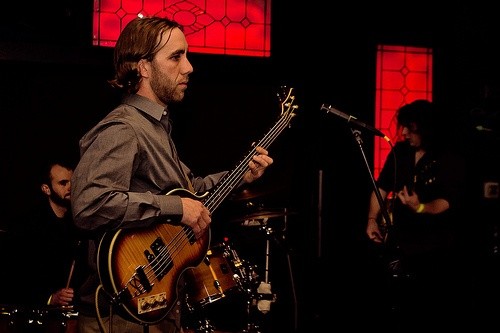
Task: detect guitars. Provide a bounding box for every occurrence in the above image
[95,83,299,326]
[377,156,438,259]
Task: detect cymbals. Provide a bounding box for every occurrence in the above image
[225,211,298,221]
[224,187,281,202]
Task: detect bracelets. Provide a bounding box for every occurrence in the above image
[416,204,425,213]
[367,217,377,219]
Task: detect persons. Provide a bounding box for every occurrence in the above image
[0,160,88,333]
[71,18,274,333]
[366,101,454,333]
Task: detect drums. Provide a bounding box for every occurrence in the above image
[79,294,147,330]
[182,243,247,307]
[44,307,79,333]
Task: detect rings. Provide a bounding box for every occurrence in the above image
[253,168,259,174]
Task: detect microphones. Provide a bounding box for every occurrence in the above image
[320,103,384,138]
